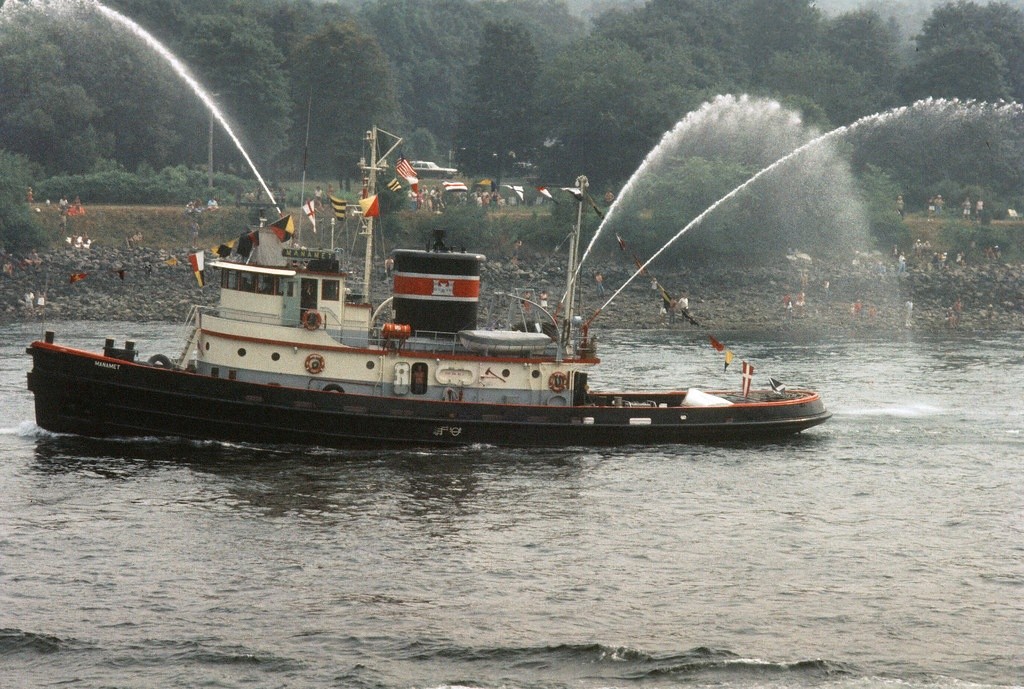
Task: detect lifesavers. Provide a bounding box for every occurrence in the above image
[304,353,325,375]
[148,353,173,369]
[321,383,345,393]
[303,309,322,331]
[548,371,568,393]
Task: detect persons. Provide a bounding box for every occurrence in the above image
[650,278,690,323]
[0,233,145,283]
[28,187,81,233]
[187,185,333,213]
[408,186,501,212]
[522,291,531,313]
[594,272,605,299]
[604,190,614,206]
[541,290,547,311]
[786,194,1001,330]
[192,221,199,248]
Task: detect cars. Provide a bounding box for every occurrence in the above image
[410,161,459,180]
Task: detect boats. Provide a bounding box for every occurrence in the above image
[457,329,554,348]
[24,86,836,458]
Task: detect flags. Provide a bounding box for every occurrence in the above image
[304,156,418,234]
[710,336,787,398]
[188,215,297,287]
